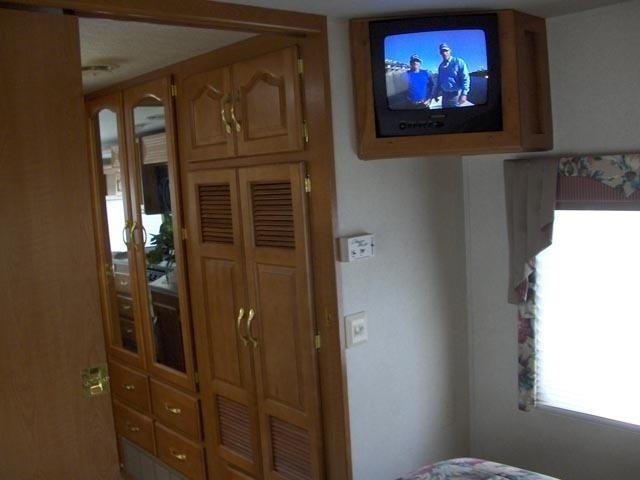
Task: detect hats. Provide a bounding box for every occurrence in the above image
[439,43,449,52]
[409,54,423,63]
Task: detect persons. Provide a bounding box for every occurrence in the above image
[402,54,436,109]
[432,42,471,108]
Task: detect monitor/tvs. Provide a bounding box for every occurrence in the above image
[369,10,503,137]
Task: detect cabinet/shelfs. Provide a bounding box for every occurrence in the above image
[175,46,304,161]
[187,163,322,479]
[107,361,206,480]
[86,77,192,391]
